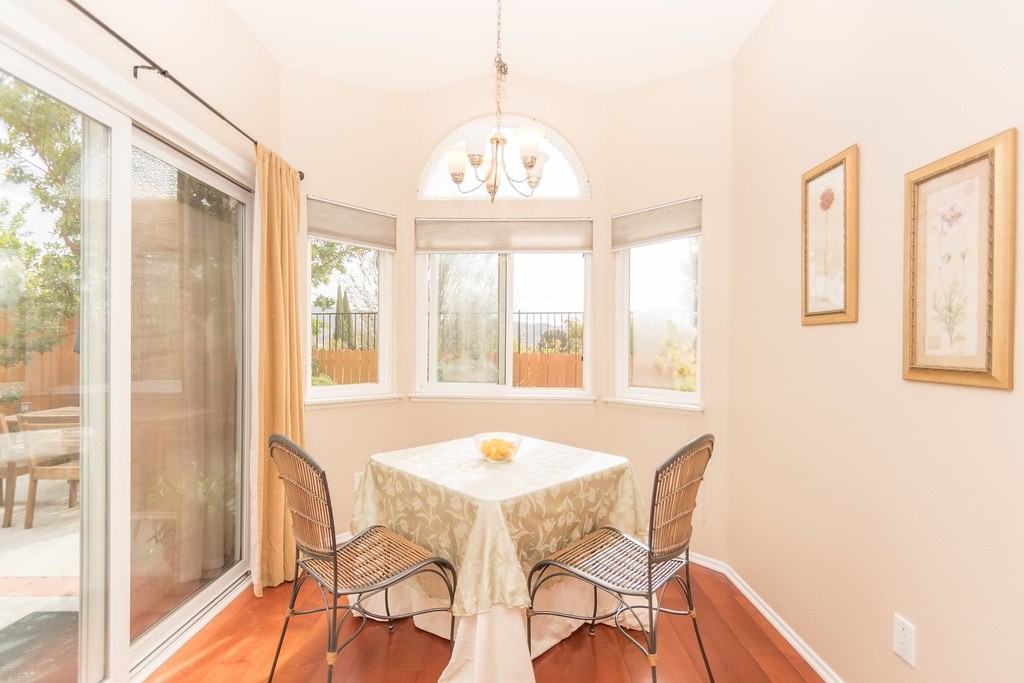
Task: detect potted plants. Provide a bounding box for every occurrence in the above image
[133,458,238,573]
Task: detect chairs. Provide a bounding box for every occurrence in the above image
[527,434,716,683]
[1,412,81,529]
[268,433,458,683]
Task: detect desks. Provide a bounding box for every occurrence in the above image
[5,407,214,433]
[345,432,659,683]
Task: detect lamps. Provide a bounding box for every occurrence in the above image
[449,1,548,204]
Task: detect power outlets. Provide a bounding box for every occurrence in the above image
[891,613,917,667]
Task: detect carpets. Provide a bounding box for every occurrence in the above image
[0,611,166,682]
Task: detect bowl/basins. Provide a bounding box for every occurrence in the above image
[474,434,523,464]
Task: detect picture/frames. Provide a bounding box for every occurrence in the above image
[901,127,1018,391]
[800,145,860,327]
